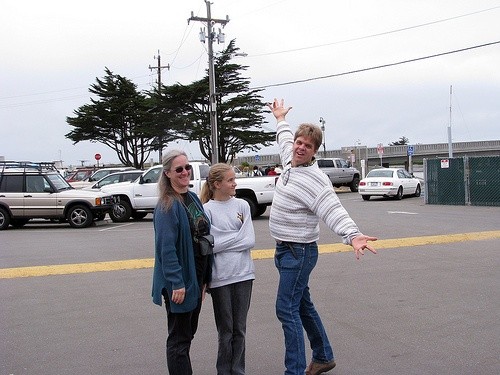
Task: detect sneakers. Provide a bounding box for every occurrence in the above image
[306,361,336,375]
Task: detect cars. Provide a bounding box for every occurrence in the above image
[55,165,147,196]
[359,167,421,200]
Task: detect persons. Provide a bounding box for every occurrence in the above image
[266,97,378,375]
[152,150,214,375]
[236,164,281,177]
[201,163,256,375]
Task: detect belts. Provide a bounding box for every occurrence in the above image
[278,241,298,259]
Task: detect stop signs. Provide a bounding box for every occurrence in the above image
[95,154,101,160]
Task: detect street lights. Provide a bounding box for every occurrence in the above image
[319,117,327,157]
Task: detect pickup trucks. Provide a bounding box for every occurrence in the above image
[100,161,281,223]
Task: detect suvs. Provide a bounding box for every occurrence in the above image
[315,158,362,192]
[0,161,114,229]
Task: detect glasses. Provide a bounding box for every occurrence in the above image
[282,168,291,186]
[170,165,192,173]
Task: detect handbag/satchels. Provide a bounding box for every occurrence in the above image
[200,235,214,255]
